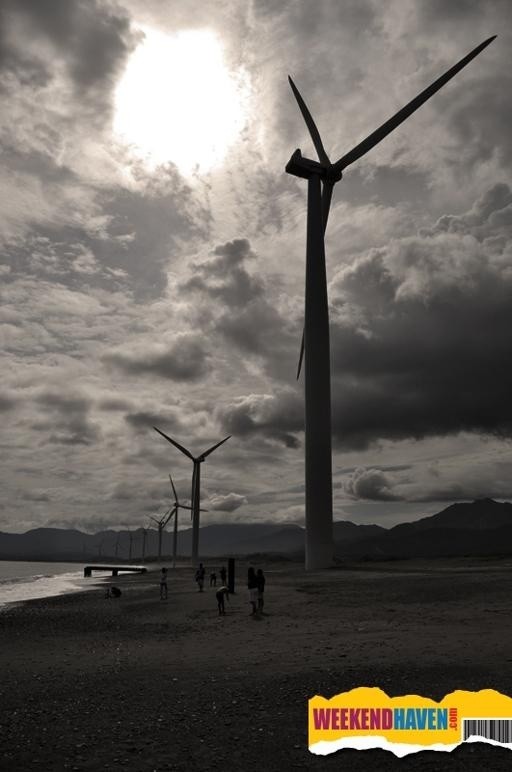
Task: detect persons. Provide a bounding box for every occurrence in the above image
[160,567,168,600]
[193,562,266,617]
